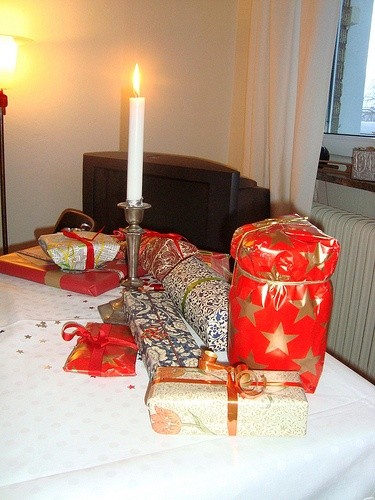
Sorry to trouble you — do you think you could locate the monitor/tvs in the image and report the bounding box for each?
[83,151,271,273]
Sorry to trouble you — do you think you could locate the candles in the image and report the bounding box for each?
[125,64,146,202]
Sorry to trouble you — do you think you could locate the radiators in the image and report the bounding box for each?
[309,202,375,384]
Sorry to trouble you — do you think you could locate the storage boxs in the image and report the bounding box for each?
[116,224,199,279]
[158,254,231,353]
[36,228,122,273]
[146,367,310,437]
[124,280,208,371]
[0,240,128,297]
[227,214,340,394]
